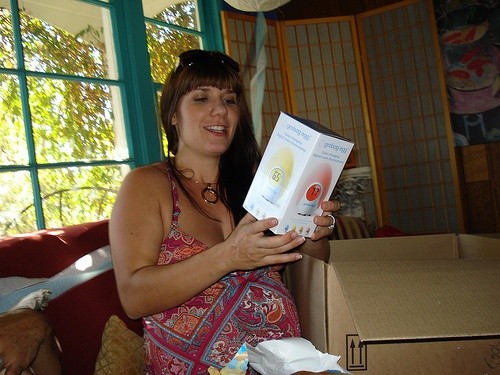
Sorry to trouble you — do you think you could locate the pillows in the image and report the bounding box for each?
[94,315,146,375]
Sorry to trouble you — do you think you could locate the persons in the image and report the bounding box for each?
[107,49,341,375]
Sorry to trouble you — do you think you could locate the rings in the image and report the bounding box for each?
[327,214,335,230]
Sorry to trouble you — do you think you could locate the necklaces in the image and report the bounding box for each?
[188,177,221,204]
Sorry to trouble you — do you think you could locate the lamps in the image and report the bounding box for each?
[224,0,289,13]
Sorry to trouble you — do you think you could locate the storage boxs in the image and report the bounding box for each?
[289,233,499,375]
[242,112,357,238]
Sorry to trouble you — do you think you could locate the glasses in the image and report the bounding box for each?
[174,49,241,81]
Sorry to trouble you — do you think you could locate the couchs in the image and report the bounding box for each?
[0,219,120,375]
